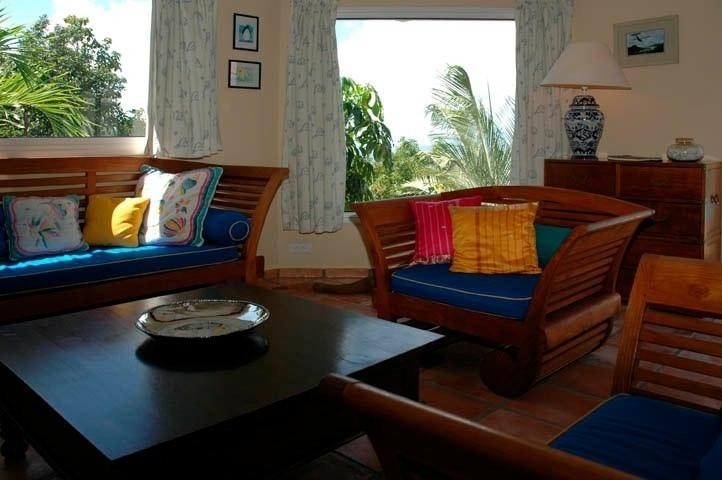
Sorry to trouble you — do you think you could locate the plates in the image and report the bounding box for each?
[134,298,273,341]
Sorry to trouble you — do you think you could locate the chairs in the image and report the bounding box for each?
[352,186,656,399]
[319,253,722,480]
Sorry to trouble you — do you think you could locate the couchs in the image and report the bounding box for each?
[0,157,289,325]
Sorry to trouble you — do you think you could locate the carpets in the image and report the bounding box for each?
[0,440,380,480]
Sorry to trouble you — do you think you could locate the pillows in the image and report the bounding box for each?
[3,163,249,262]
[409,196,572,274]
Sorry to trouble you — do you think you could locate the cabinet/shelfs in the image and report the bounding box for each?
[544,159,722,317]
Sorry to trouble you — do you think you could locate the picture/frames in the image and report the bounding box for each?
[229,59,261,89]
[613,15,679,68]
[233,13,259,52]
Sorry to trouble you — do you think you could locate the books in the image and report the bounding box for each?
[608,154,663,163]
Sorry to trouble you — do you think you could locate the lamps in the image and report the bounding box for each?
[541,41,631,161]
